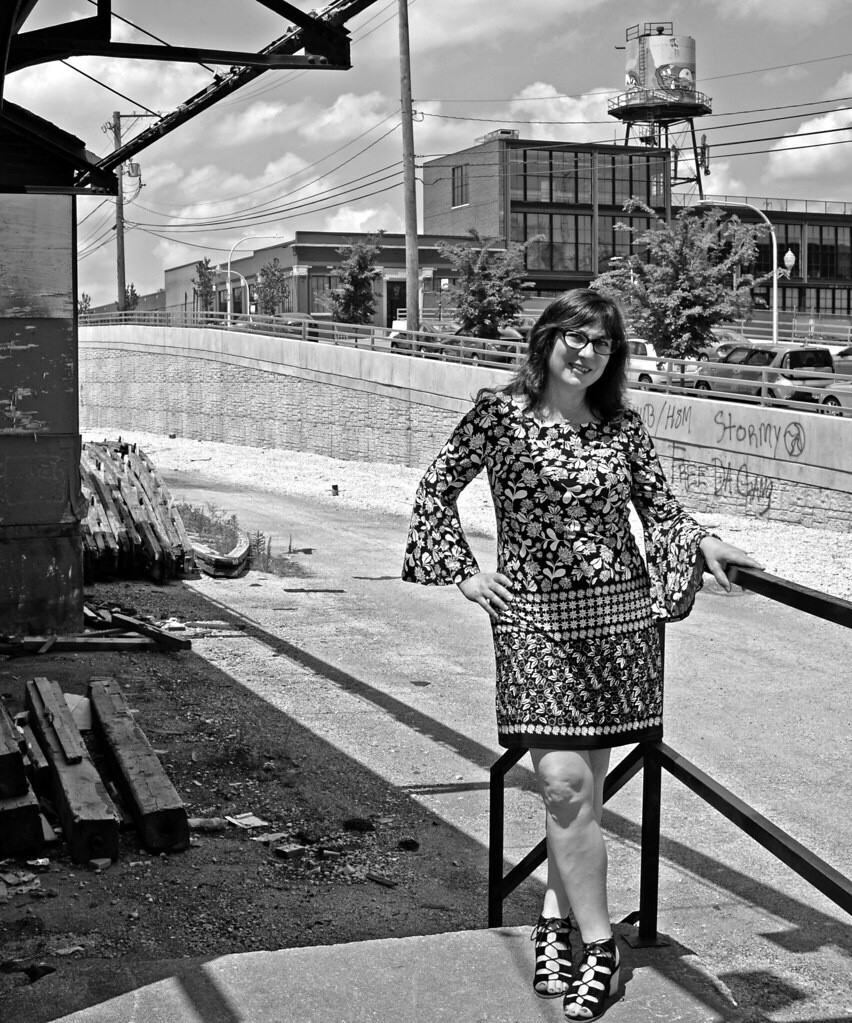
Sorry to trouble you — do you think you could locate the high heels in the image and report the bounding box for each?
[562,937,620,1022]
[532,911,572,999]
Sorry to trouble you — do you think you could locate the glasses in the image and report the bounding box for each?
[555,328,622,355]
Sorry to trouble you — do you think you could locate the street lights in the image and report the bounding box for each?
[696,198,795,344]
[228,233,285,324]
[208,263,250,327]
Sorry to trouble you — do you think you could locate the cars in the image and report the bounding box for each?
[624,328,852,417]
[232,312,321,342]
[392,318,538,371]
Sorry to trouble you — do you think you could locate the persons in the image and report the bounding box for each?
[401,288,766,1023]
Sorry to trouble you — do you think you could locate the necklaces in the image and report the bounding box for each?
[556,402,585,425]
